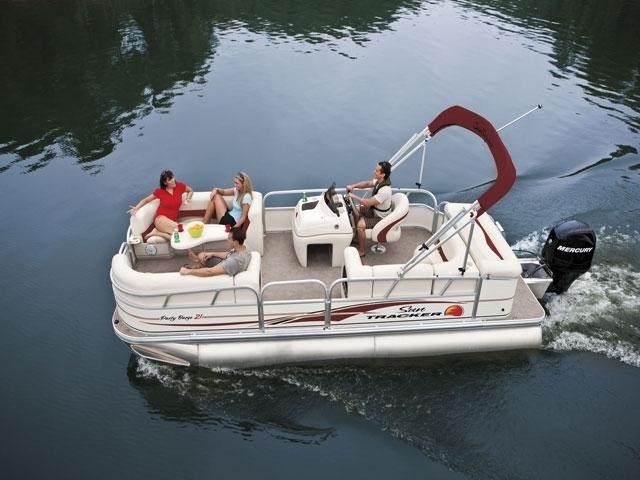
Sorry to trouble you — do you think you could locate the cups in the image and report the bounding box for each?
[178,224,183,232]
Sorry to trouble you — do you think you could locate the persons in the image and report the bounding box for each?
[203,171,252,227]
[346,161,392,257]
[126,170,200,263]
[180,227,250,277]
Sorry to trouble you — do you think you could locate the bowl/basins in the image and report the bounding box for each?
[187,221,204,238]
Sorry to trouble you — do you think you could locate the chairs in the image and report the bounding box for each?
[364,192,410,255]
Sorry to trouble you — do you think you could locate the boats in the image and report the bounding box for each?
[109,103,596,372]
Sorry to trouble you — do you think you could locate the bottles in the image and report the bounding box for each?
[173,229,180,243]
[302,192,308,202]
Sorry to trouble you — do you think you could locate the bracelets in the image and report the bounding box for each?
[186,197,190,200]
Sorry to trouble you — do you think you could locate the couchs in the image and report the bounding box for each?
[110,190,264,302]
[338,229,480,298]
